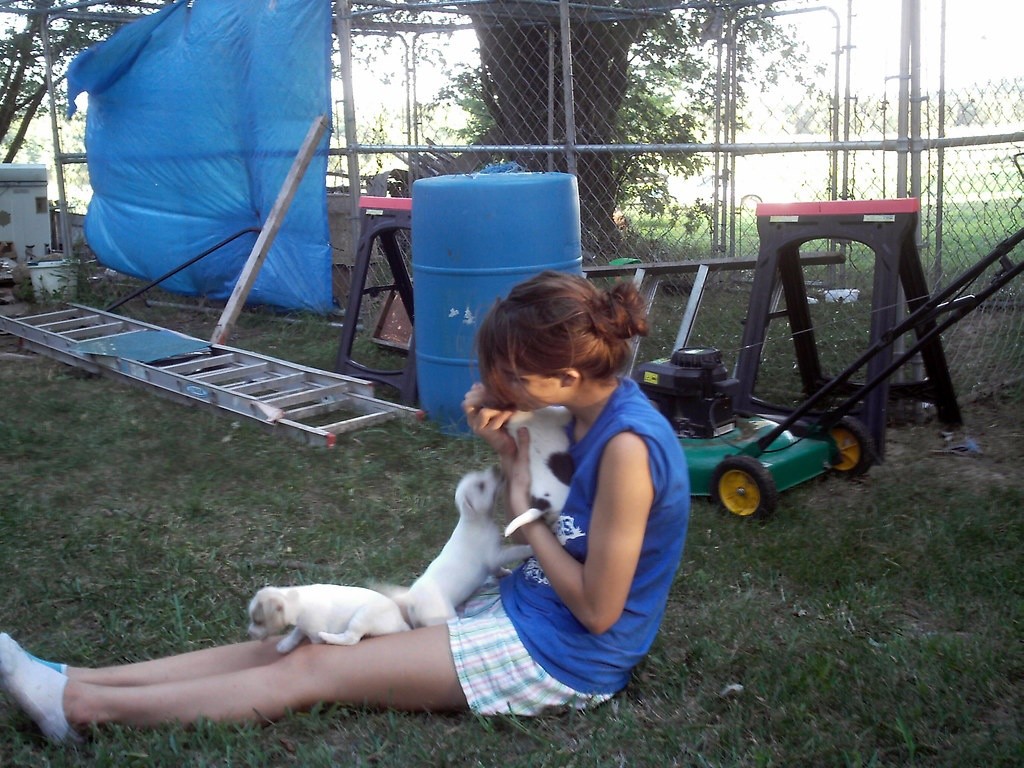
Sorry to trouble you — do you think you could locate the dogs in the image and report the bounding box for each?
[24,245,37,261]
[368,464,533,628]
[246,583,411,653]
[464,379,573,537]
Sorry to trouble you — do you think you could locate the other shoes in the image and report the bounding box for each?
[0,631,85,749]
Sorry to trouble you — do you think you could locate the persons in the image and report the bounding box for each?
[1,268,692,750]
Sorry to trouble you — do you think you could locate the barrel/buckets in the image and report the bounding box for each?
[413,172,581,436]
[28,260,77,303]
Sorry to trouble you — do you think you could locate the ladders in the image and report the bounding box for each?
[0,301,424,448]
[368,252,847,435]
